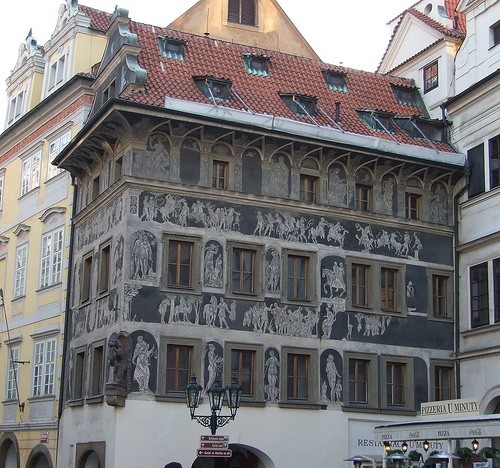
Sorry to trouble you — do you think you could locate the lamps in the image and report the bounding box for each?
[423,440,429,453]
[402,440,408,454]
[384,441,390,452]
[472,439,479,453]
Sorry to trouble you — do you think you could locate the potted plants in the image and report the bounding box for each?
[408,450,424,465]
[478,446,500,462]
[457,447,473,458]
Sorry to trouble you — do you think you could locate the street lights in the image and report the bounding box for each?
[184,370,241,468]
